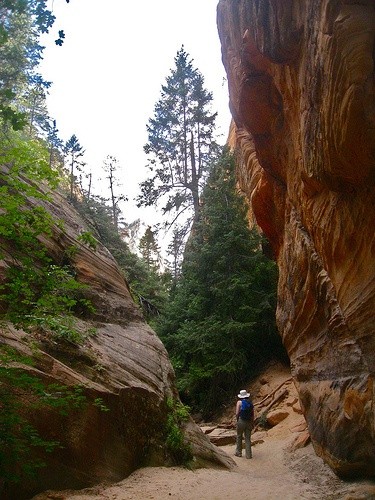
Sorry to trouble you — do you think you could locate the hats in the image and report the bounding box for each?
[237,390,250,397]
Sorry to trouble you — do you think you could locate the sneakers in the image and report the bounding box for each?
[234,452,242,457]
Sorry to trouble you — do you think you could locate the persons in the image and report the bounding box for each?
[234,390,254,459]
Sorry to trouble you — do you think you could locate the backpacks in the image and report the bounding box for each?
[240,401,254,420]
